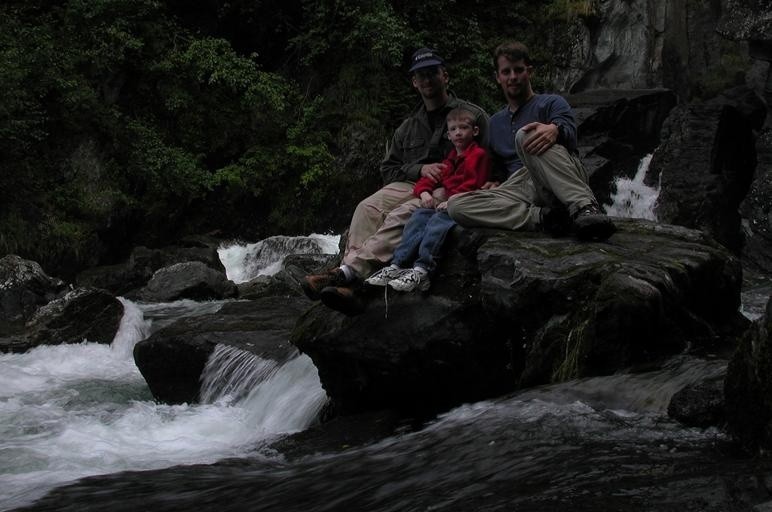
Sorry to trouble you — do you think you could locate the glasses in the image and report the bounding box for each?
[413,69,438,80]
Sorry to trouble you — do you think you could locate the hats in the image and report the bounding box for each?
[408,48,445,73]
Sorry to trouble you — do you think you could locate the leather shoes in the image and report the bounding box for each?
[300,266,360,316]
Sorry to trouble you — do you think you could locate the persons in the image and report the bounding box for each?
[362,106,492,295]
[301,46,490,318]
[446,38,618,243]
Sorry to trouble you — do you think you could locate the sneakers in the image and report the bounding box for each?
[363,266,431,292]
[575,204,611,237]
